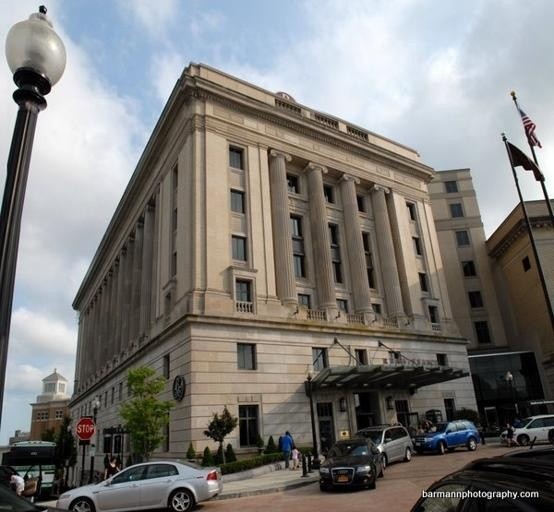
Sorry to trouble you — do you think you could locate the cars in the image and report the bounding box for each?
[319,436,384,492]
[500,414,554,447]
[0,458,224,512]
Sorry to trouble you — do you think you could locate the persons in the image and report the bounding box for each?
[281,431,292,468]
[8,470,25,497]
[505,421,521,448]
[103,456,121,480]
[292,447,301,469]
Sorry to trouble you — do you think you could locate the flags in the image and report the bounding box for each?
[505,139,545,182]
[519,105,542,148]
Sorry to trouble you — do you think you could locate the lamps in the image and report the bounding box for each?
[387,396,394,410]
[339,398,347,412]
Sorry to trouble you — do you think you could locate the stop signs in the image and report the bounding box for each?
[76,418,95,442]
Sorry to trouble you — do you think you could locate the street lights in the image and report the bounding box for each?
[0,3,68,430]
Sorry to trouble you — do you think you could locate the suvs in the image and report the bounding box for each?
[352,423,414,470]
[411,418,482,455]
[409,436,554,511]
